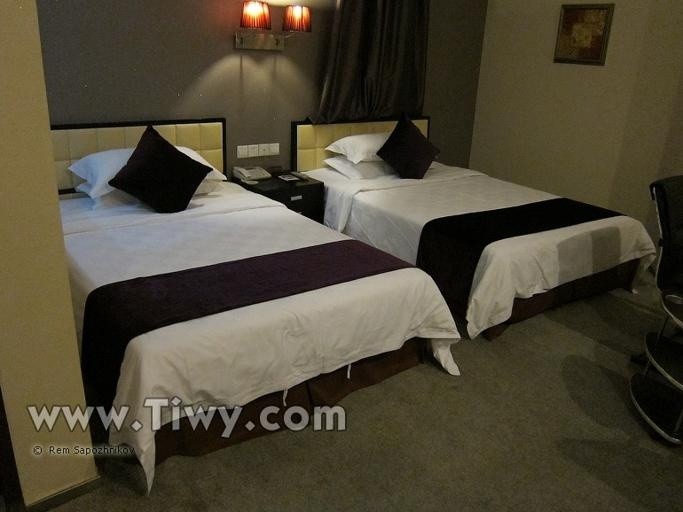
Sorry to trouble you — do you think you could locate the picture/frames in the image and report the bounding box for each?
[551,3,613,68]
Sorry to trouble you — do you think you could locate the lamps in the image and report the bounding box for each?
[239,2,313,50]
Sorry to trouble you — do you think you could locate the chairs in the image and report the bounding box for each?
[627,171,682,449]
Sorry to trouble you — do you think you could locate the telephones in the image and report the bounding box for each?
[232,166,272,185]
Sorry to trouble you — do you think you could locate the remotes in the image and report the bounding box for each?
[291,170,308,182]
